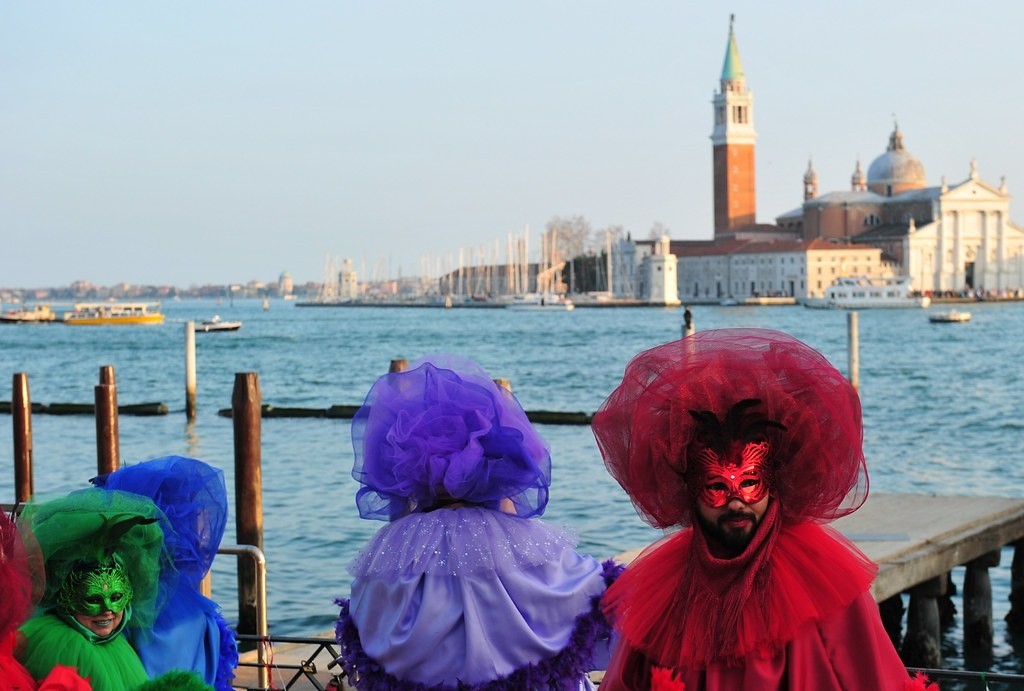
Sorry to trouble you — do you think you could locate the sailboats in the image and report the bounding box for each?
[433,230,576,313]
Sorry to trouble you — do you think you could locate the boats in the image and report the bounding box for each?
[194,320,243,332]
[61,298,166,326]
[802,272,931,311]
[928,308,973,324]
[0,301,56,323]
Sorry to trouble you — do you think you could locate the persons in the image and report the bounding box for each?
[88,456,239,691]
[584,331,940,691]
[15,487,217,691]
[333,354,627,690]
[684,305,692,329]
